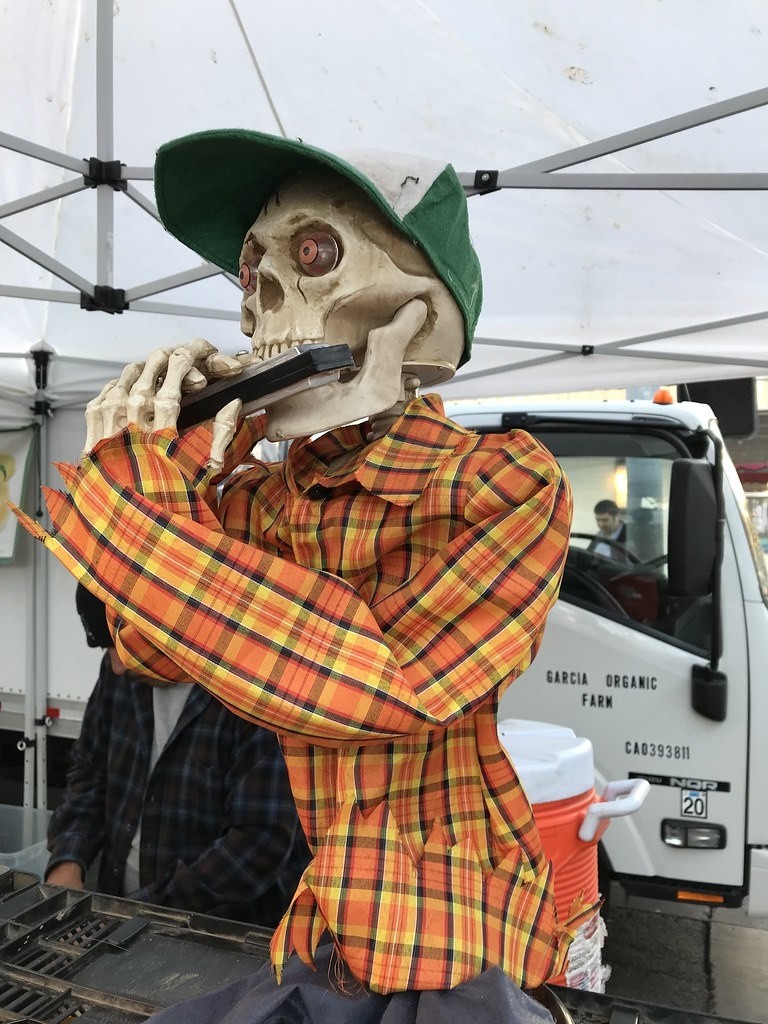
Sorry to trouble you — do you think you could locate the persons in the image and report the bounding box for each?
[43,569,313,929]
[586,500,627,562]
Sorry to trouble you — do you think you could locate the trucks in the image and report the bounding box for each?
[1,384,767,926]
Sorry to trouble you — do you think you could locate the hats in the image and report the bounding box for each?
[151,127,491,365]
